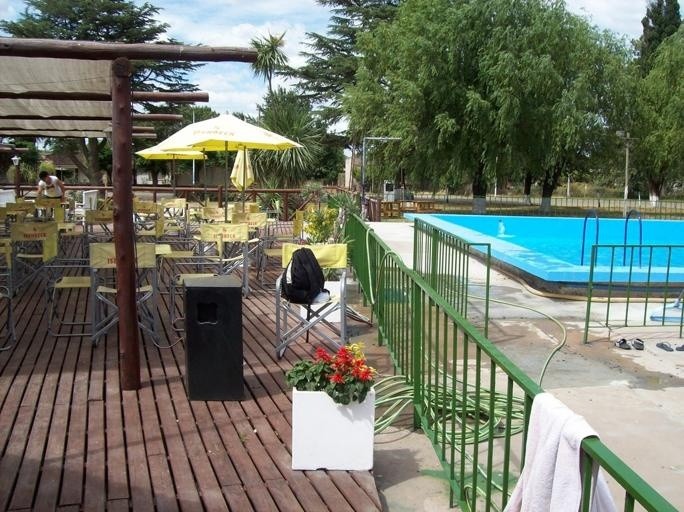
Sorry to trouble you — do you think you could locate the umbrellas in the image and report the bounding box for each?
[160,110,305,265]
[134,145,208,216]
[230,149,254,192]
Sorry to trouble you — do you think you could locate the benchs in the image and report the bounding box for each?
[380,200,442,219]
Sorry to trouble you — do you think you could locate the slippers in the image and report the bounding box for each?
[632,339,644,350]
[676,344,684,351]
[615,338,630,349]
[656,342,673,351]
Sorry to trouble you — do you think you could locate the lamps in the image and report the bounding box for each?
[11,155,22,165]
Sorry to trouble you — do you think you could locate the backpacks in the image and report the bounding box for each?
[281,248,331,343]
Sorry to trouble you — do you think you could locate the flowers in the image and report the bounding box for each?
[285,341,378,405]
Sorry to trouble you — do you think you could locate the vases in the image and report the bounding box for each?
[292,386,375,470]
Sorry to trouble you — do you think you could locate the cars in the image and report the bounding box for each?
[665,179,684,197]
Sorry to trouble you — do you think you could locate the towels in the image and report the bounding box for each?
[503,393,617,512]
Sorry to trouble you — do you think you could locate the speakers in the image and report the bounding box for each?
[181,276,243,401]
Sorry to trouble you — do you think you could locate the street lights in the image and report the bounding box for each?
[367,143,379,194]
[11,153,23,200]
[615,129,630,200]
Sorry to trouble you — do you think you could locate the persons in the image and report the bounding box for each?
[37,171,65,216]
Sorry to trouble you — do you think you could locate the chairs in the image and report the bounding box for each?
[261,203,347,360]
[2,194,274,351]
